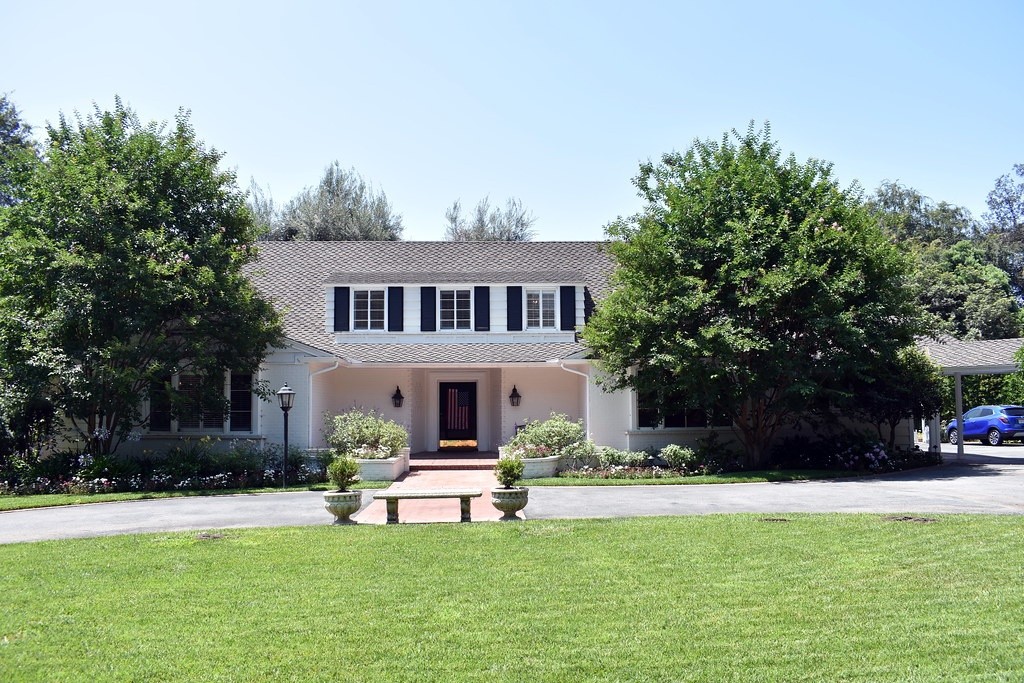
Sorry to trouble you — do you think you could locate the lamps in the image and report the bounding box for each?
[391,385,404,408]
[509,385,522,406]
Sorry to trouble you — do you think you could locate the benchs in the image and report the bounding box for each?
[372,490,482,524]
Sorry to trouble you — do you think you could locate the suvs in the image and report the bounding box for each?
[946,405,1024,445]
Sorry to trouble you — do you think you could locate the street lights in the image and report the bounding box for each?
[276,381,296,488]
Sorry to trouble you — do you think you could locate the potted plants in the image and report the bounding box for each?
[490,455,529,520]
[323,454,363,525]
